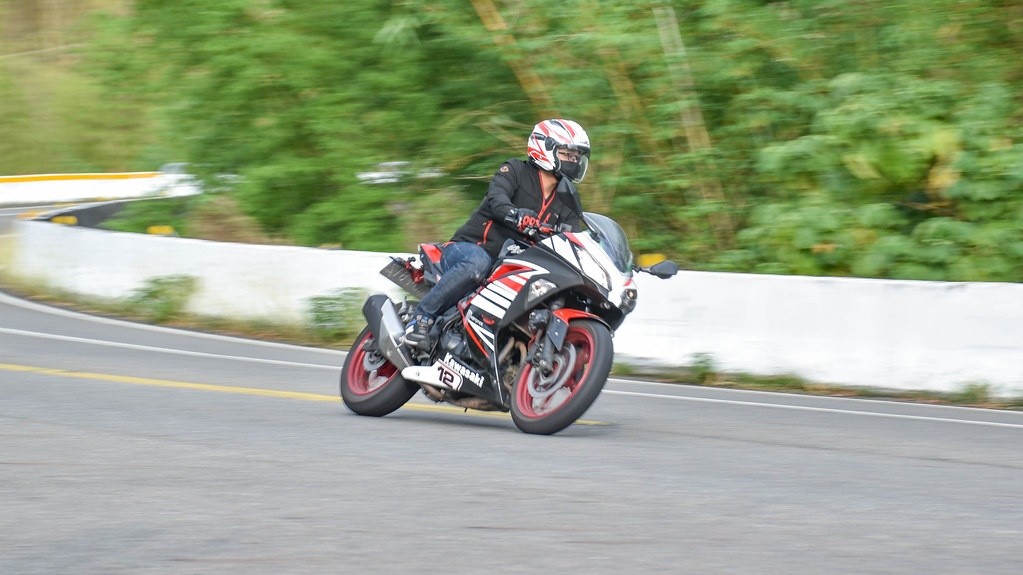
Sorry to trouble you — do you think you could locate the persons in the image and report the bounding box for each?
[403,119,591,352]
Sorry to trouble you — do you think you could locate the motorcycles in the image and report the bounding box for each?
[339,177,681,436]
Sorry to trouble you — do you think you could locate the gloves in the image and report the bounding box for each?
[504,207,541,237]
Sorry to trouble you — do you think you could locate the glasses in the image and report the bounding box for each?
[558,151,582,165]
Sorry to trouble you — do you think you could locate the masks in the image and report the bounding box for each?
[553,160,580,182]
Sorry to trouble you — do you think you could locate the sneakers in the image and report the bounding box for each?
[404,314,433,351]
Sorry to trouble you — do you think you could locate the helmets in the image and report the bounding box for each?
[528,119,590,184]
